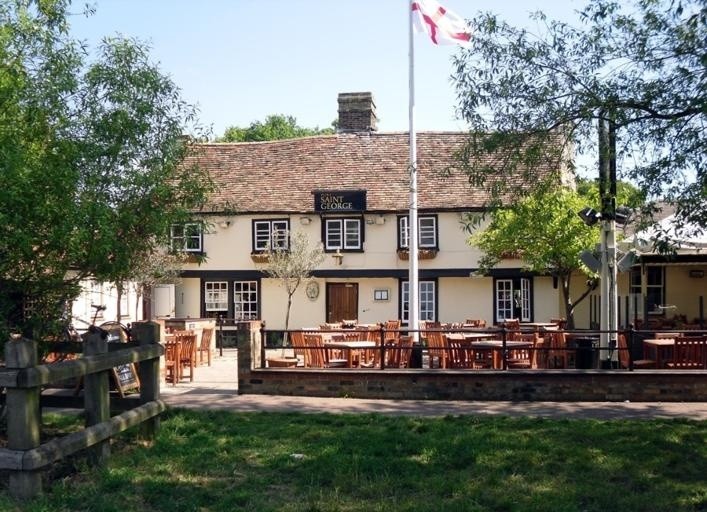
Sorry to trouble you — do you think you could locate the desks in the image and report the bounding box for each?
[578,191,636,371]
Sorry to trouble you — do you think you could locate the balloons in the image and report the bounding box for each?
[305,281,319,298]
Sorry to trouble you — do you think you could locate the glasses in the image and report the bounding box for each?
[300,217,312,225]
[218,221,229,227]
[375,216,385,225]
[332,247,343,265]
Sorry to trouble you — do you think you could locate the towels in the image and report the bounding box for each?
[406,342,423,368]
[574,337,600,369]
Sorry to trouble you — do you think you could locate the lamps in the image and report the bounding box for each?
[165,327,214,386]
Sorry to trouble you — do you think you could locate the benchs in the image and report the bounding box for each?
[412,1,479,47]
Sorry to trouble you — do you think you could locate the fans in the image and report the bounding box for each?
[99,325,140,392]
